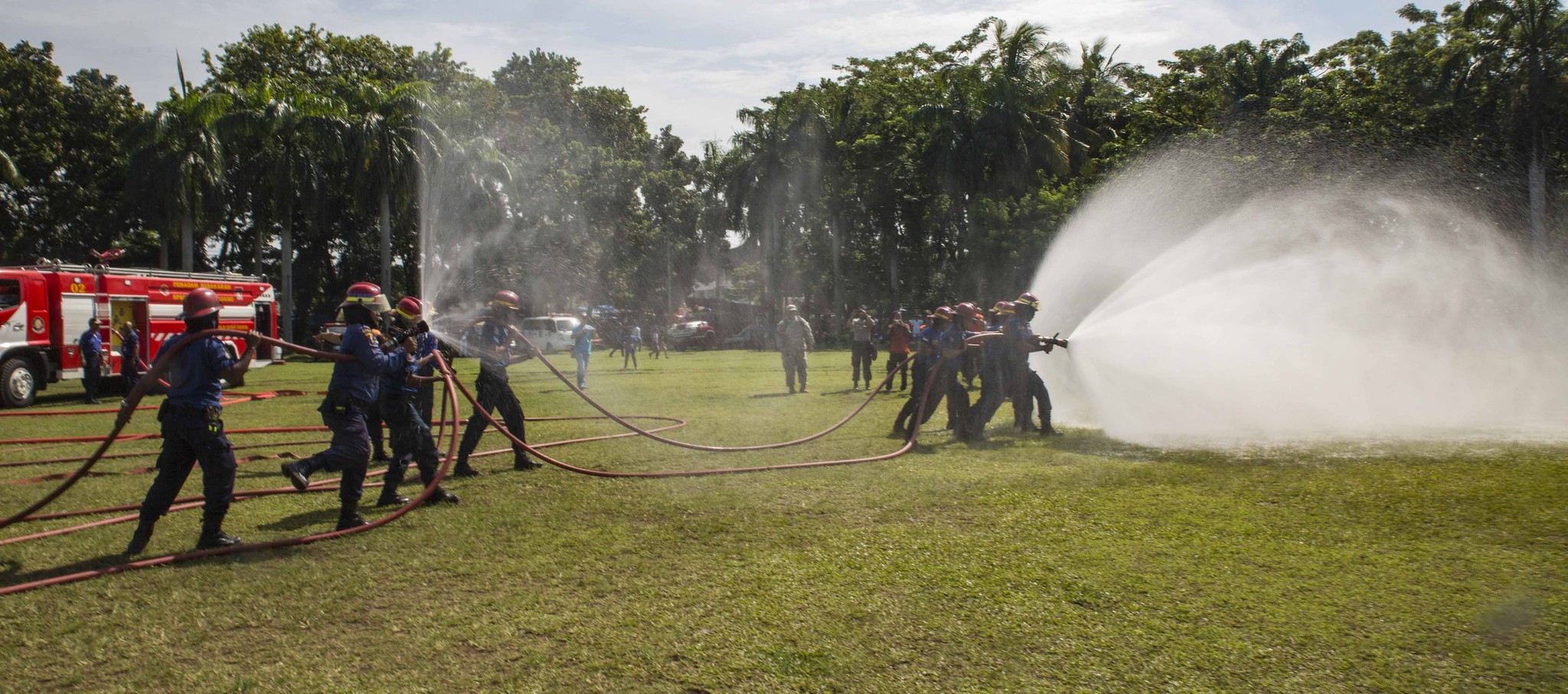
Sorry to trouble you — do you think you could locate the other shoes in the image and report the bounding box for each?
[87,399,102,404]
[853,386,858,390]
[515,454,542,470]
[376,492,409,505]
[279,460,310,490]
[865,385,871,389]
[129,532,148,553]
[373,451,392,461]
[454,463,479,476]
[789,389,796,394]
[195,529,242,548]
[336,513,367,530]
[425,490,459,504]
[888,419,1063,447]
[579,385,586,390]
[800,387,808,392]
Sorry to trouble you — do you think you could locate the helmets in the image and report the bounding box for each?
[419,299,438,315]
[388,296,423,320]
[176,288,224,320]
[488,290,520,310]
[926,292,1038,322]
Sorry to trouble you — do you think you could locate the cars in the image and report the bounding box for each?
[324,323,347,361]
[723,325,774,350]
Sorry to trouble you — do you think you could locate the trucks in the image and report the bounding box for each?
[667,306,717,351]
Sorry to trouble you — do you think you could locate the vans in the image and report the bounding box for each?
[518,312,581,353]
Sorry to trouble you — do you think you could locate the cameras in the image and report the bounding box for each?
[588,329,592,331]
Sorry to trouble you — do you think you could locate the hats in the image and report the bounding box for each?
[786,305,797,311]
[581,312,587,317]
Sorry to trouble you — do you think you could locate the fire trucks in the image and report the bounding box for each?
[0,248,290,409]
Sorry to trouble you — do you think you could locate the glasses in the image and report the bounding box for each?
[337,282,384,310]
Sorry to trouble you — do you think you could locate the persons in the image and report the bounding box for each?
[751,291,1065,441]
[74,277,668,562]
